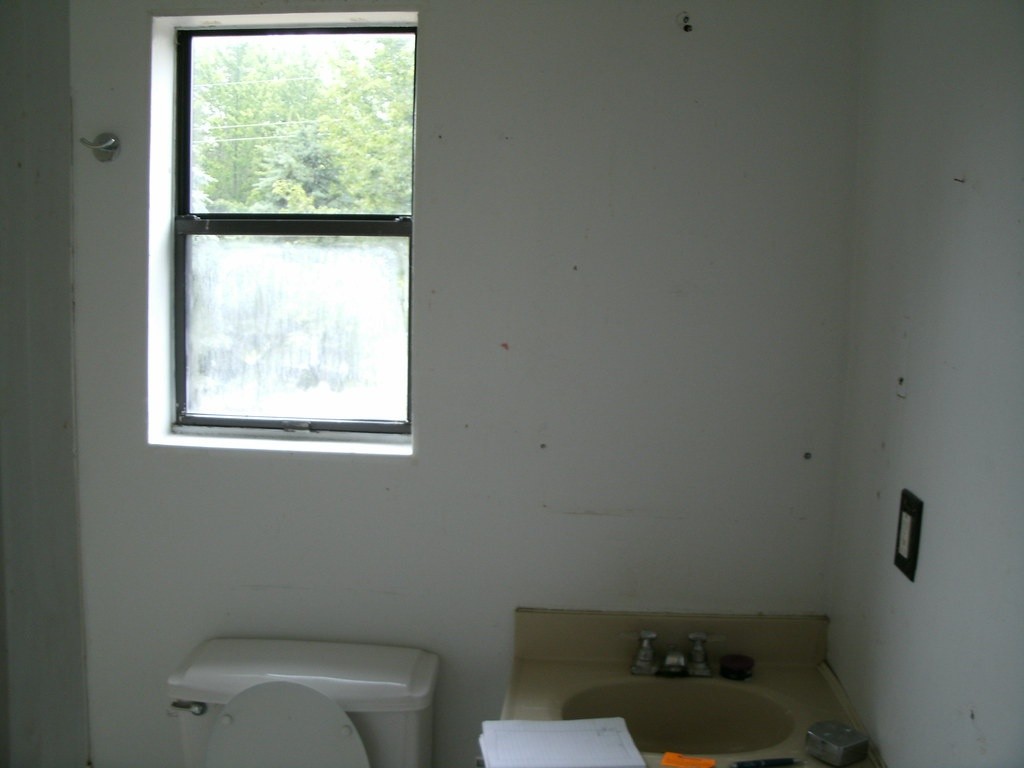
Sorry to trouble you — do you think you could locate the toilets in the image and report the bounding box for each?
[166,637,442,768]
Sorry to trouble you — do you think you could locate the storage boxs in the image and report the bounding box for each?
[805,719,871,768]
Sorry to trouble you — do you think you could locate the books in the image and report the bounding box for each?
[480,717,646,768]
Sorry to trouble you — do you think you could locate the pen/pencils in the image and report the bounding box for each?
[731,758,804,768]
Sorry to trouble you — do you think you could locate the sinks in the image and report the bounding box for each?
[557,677,795,756]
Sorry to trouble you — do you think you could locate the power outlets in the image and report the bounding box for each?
[891,485,923,584]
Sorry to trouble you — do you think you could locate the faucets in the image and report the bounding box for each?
[655,641,690,673]
